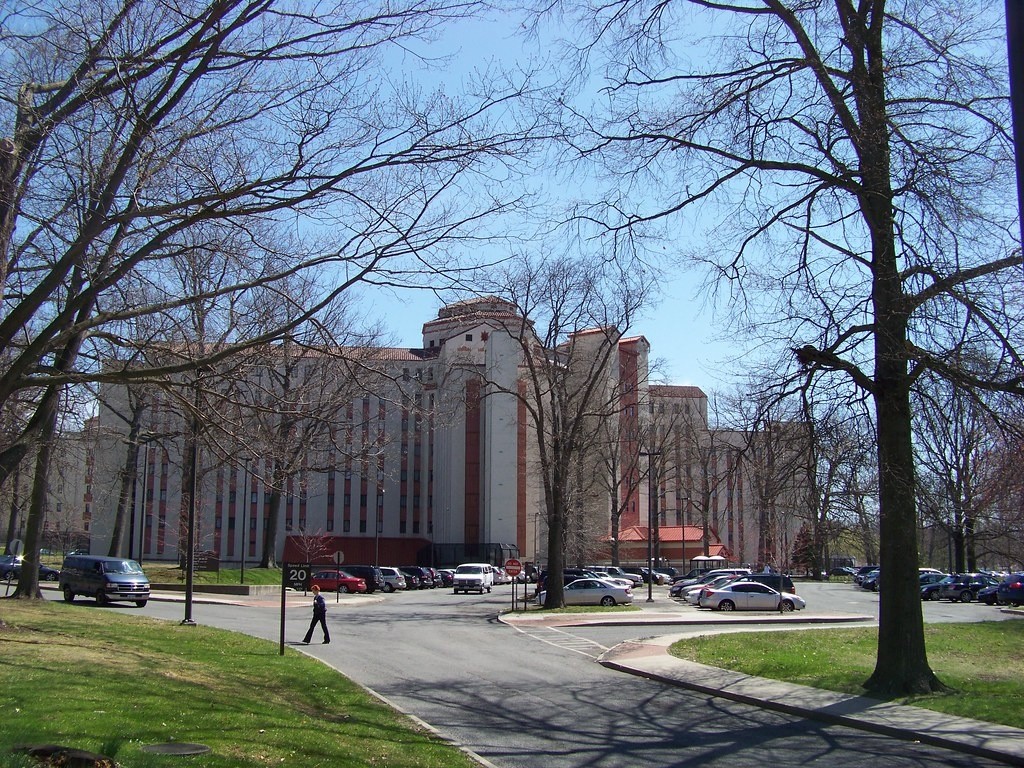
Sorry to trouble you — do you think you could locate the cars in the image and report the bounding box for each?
[39,548,53,556]
[827,566,1024,606]
[437,569,456,587]
[537,564,679,612]
[491,565,542,585]
[0,554,60,582]
[400,571,420,590]
[669,566,806,611]
[66,546,89,555]
[307,569,367,593]
[426,567,442,589]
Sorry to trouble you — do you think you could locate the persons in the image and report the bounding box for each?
[300,585,331,645]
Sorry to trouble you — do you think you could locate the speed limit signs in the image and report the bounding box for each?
[284,563,311,587]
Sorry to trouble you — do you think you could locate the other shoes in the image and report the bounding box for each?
[303,640,309,644]
[322,641,329,644]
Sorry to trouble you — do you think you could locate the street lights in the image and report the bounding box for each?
[676,498,690,576]
[639,451,662,602]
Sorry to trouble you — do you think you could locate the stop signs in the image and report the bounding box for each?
[506,560,521,575]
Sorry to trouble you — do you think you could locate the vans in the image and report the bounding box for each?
[399,566,433,589]
[339,565,385,594]
[58,554,150,608]
[379,566,406,593]
[453,563,493,595]
